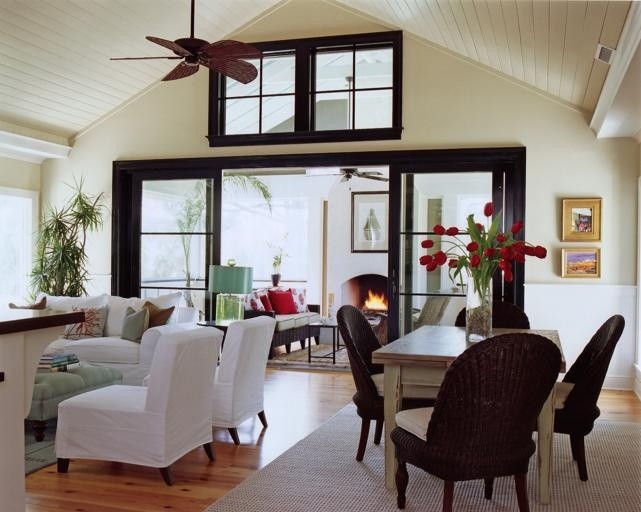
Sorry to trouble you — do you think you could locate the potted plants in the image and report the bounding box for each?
[268,247,284,287]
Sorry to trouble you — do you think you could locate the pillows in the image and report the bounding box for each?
[244,288,306,315]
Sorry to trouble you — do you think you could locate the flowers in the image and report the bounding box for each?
[417,196,548,307]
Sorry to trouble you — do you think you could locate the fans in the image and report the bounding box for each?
[305,76,390,187]
[110,0,263,84]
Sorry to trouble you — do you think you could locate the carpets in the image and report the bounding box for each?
[204,390,641,511]
[264,339,352,372]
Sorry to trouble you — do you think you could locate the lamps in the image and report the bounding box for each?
[204,260,257,325]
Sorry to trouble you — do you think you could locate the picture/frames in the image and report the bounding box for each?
[561,248,602,279]
[349,191,389,254]
[561,198,603,241]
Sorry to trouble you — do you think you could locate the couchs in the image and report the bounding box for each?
[238,287,323,362]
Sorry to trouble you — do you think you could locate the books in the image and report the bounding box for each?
[36,353,81,372]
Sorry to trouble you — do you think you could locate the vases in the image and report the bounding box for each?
[463,274,497,342]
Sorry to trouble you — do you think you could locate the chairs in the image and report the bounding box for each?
[391,335,563,511]
[454,306,531,330]
[411,293,453,330]
[524,314,627,484]
[337,303,437,462]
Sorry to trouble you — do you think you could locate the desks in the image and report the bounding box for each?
[198,319,233,340]
[368,326,570,509]
[0,304,88,510]
